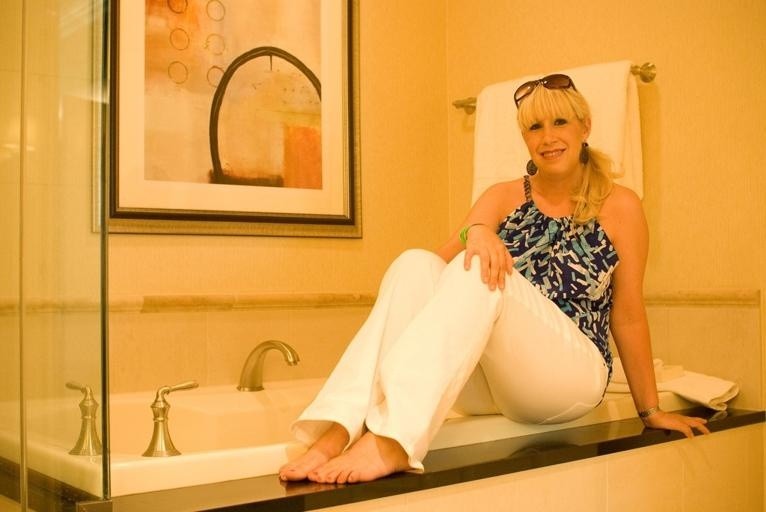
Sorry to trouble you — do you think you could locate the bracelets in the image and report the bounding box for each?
[639,404,661,418]
[459,223,485,245]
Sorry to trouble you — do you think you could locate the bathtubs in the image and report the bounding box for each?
[0,378,702,497]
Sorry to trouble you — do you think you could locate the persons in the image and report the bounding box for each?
[279,75,711,484]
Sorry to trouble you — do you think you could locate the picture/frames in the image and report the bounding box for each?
[93,0,363,237]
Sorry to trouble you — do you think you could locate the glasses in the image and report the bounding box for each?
[514,74,577,108]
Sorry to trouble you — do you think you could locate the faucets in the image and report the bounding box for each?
[236,340,300,393]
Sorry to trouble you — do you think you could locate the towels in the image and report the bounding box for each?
[473,63,645,208]
[605,356,740,412]
